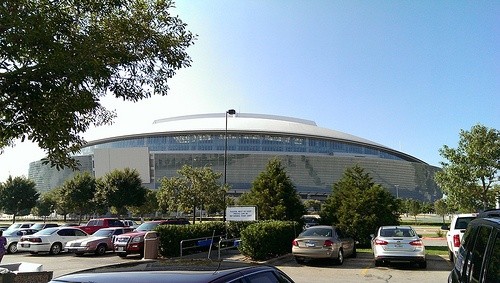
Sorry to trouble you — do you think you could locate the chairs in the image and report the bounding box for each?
[403,231,411,237]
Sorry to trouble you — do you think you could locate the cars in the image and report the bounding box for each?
[0,219,141,256]
[0,229,7,262]
[44,258,298,283]
[291,225,357,265]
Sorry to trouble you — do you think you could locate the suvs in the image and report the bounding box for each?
[111,218,191,258]
[369,224,427,269]
[447,209,500,283]
[441,213,480,263]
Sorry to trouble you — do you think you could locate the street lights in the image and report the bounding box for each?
[394,184,400,200]
[223,109,237,227]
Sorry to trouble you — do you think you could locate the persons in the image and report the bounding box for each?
[0,231,7,262]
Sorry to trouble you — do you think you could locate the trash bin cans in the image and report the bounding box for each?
[143,231,160,259]
